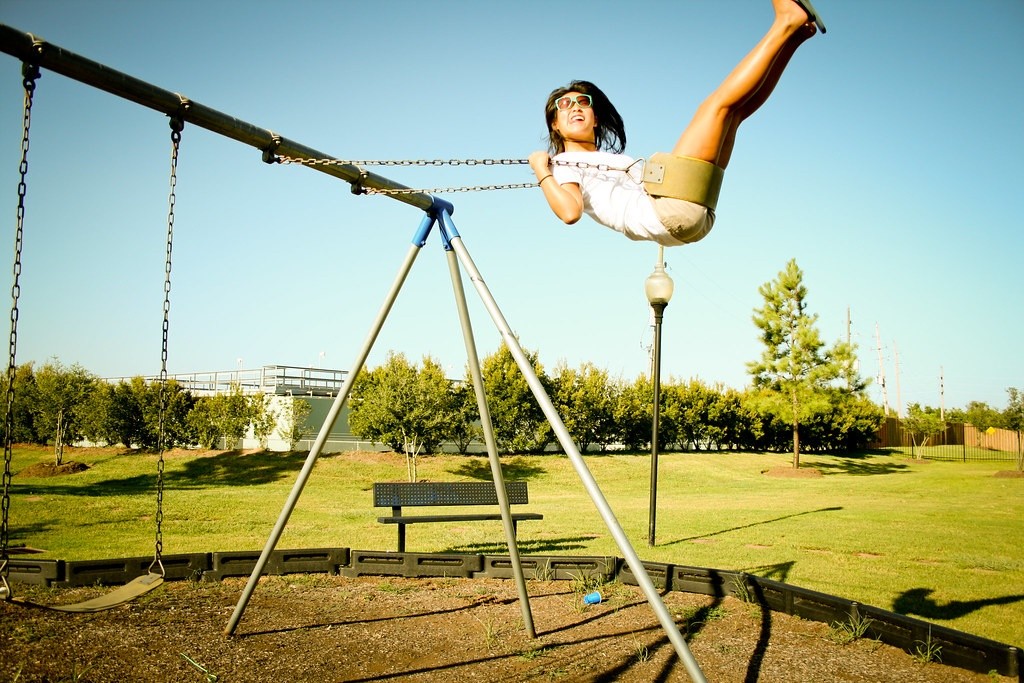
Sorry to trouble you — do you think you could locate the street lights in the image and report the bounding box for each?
[646,243,674,544]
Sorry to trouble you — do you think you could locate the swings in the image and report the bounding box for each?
[0,74,182,614]
[276,154,727,210]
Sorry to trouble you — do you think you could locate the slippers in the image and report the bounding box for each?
[814,10,826,34]
[794,0,815,22]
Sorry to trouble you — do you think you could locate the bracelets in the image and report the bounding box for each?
[539,175,552,187]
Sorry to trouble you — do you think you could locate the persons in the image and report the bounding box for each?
[528,0,825,247]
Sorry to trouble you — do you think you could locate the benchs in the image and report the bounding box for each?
[372,482,542,552]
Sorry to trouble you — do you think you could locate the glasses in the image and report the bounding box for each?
[553,94,594,111]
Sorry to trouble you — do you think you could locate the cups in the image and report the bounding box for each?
[584,592,602,604]
